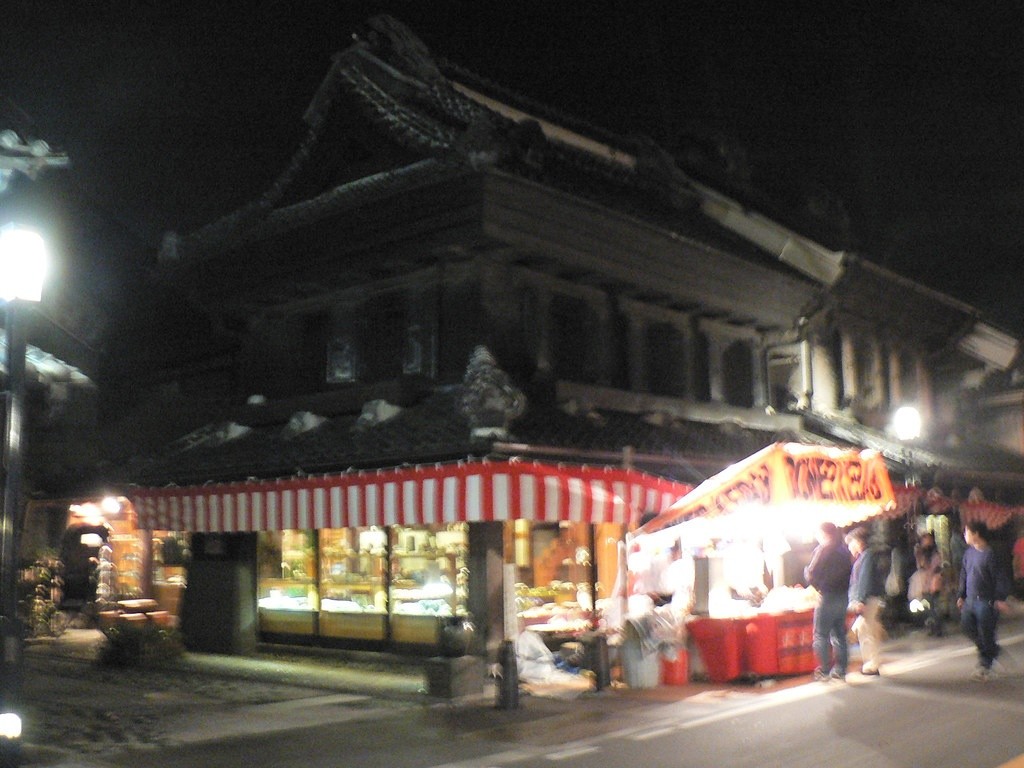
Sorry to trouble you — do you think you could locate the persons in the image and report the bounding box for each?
[1011,538,1024,581]
[844,528,885,676]
[804,522,852,683]
[914,533,943,637]
[956,521,1007,685]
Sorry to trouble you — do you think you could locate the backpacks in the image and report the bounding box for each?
[885,547,904,597]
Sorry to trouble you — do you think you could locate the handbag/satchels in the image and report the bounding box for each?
[851,612,868,636]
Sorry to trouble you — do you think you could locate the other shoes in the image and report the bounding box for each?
[828,665,846,679]
[927,623,943,638]
[860,662,880,675]
[972,665,990,683]
[813,667,833,682]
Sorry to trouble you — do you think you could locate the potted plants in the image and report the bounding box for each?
[618,620,664,689]
